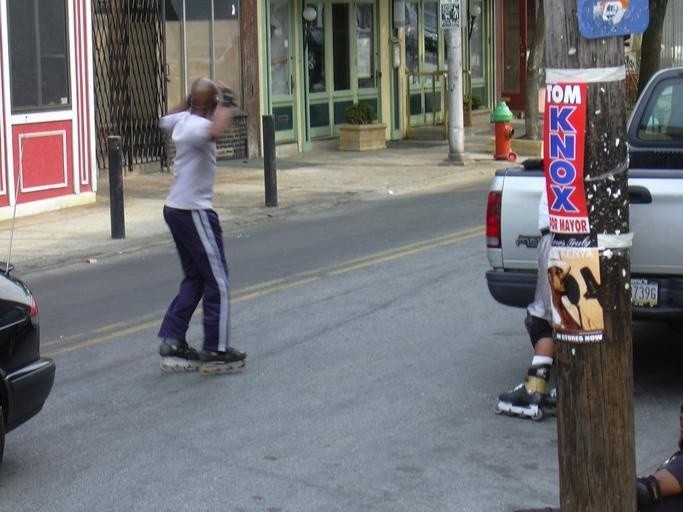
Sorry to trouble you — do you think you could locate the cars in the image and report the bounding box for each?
[405,9,437,53]
[0,260,56,465]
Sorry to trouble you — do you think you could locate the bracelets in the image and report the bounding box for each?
[221,95,233,108]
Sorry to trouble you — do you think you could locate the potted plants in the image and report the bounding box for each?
[336,102,388,152]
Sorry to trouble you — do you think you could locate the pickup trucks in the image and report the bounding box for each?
[484,65,683,322]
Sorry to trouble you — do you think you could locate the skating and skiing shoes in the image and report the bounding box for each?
[158,339,247,373]
[494,362,551,421]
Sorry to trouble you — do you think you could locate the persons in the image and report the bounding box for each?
[450,3,458,23]
[497,186,555,408]
[156,75,249,364]
[634,398,682,512]
[547,249,586,332]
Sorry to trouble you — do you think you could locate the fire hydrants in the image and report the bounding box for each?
[490,101,517,161]
